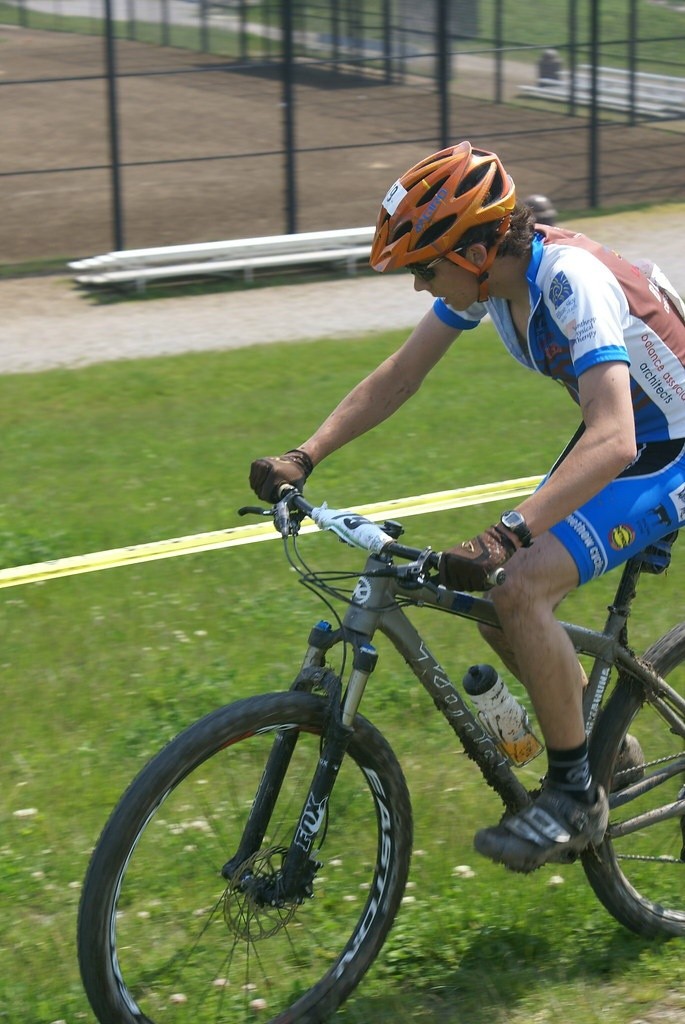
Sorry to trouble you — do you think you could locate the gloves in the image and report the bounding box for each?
[249,450,314,504]
[434,525,517,593]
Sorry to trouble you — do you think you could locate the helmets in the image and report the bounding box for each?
[368,141,516,273]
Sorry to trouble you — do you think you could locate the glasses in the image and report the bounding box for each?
[403,241,487,282]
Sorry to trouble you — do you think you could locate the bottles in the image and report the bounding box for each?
[463,664,539,763]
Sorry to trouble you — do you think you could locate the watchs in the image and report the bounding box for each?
[500,510,535,548]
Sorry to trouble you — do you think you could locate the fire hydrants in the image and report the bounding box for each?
[538,50,563,89]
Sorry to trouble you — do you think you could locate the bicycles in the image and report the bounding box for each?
[73,467,684,1024]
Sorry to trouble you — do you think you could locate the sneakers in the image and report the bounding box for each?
[474,784,610,872]
[609,732,646,792]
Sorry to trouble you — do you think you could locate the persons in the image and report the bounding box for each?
[538,48,561,87]
[249,139,685,874]
